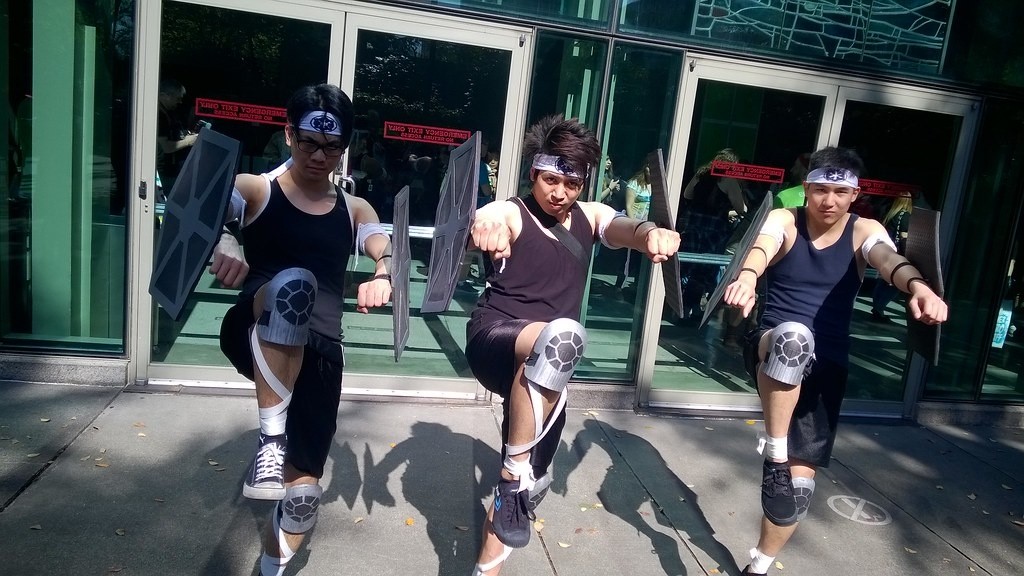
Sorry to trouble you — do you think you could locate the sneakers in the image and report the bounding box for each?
[760,457,798,528]
[742,565,768,576]
[492,477,537,548]
[242,436,289,500]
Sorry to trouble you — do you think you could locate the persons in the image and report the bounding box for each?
[209,83,393,576]
[723,147,947,576]
[155,79,914,348]
[466,113,681,576]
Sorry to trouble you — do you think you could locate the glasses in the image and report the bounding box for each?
[289,126,346,158]
